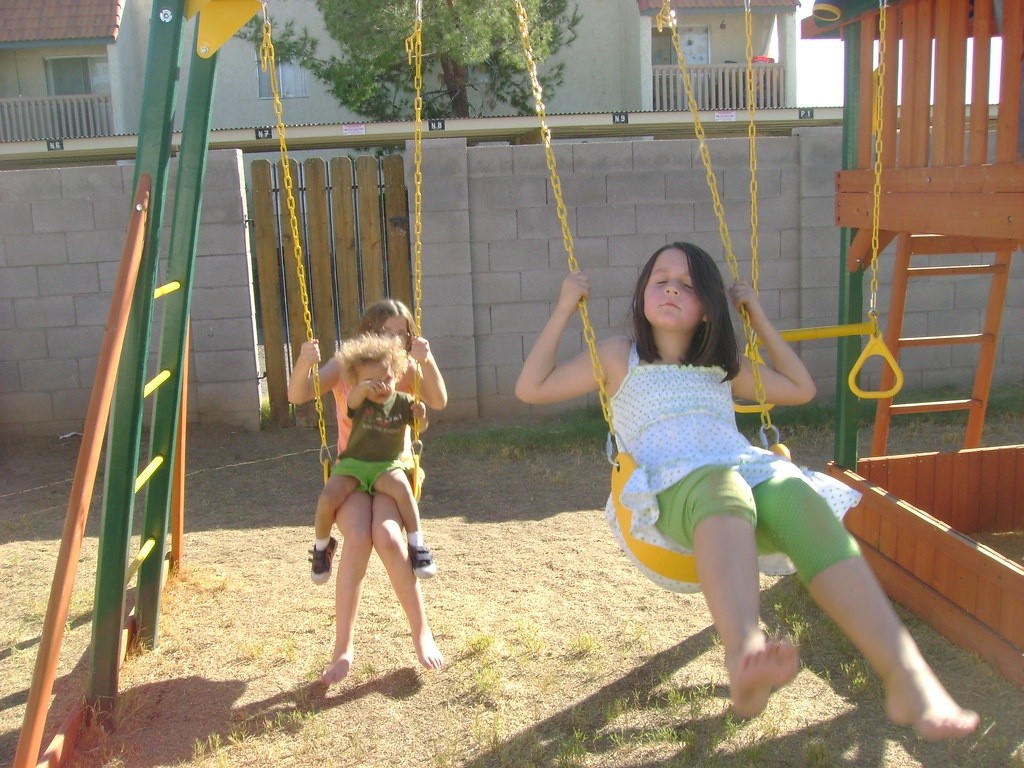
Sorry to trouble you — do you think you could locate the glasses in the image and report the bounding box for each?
[374,326,410,340]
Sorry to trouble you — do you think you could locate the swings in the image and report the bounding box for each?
[261,18,424,536]
[747,0,890,343]
[515,0,791,585]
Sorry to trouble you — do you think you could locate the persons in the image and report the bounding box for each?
[308,329,437,586]
[514,242,980,740]
[287,299,447,681]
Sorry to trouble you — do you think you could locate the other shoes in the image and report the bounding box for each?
[307,536,338,583]
[408,543,436,579]
